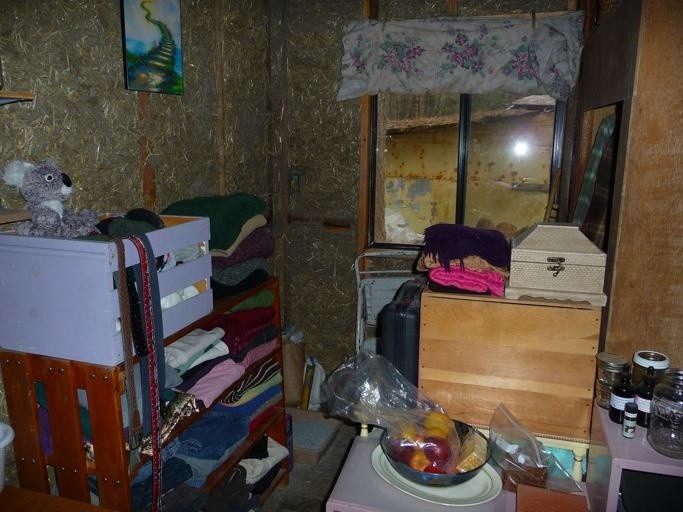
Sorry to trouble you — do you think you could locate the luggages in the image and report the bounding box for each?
[375,276,427,410]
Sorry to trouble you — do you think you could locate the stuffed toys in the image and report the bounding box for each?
[2,160,101,239]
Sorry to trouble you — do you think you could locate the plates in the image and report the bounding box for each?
[370,443,504,506]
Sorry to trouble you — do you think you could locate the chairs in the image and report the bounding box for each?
[348,244,423,367]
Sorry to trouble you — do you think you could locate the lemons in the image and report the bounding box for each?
[424,410,450,439]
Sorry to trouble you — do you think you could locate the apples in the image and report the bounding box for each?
[409,450,430,472]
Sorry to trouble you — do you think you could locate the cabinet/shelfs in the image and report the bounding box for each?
[0,269,297,512]
[319,399,681,512]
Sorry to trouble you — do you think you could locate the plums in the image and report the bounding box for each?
[390,439,414,461]
[424,437,451,462]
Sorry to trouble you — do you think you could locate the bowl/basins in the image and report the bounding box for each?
[379,418,490,485]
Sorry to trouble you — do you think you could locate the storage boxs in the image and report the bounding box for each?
[0,211,215,375]
[500,220,610,297]
[414,279,605,449]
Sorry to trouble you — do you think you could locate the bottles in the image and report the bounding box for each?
[635,366,656,429]
[594,353,630,407]
[621,402,639,438]
[646,369,682,460]
[631,350,671,389]
[609,364,636,424]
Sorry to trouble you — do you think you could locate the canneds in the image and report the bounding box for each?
[596,350,670,410]
